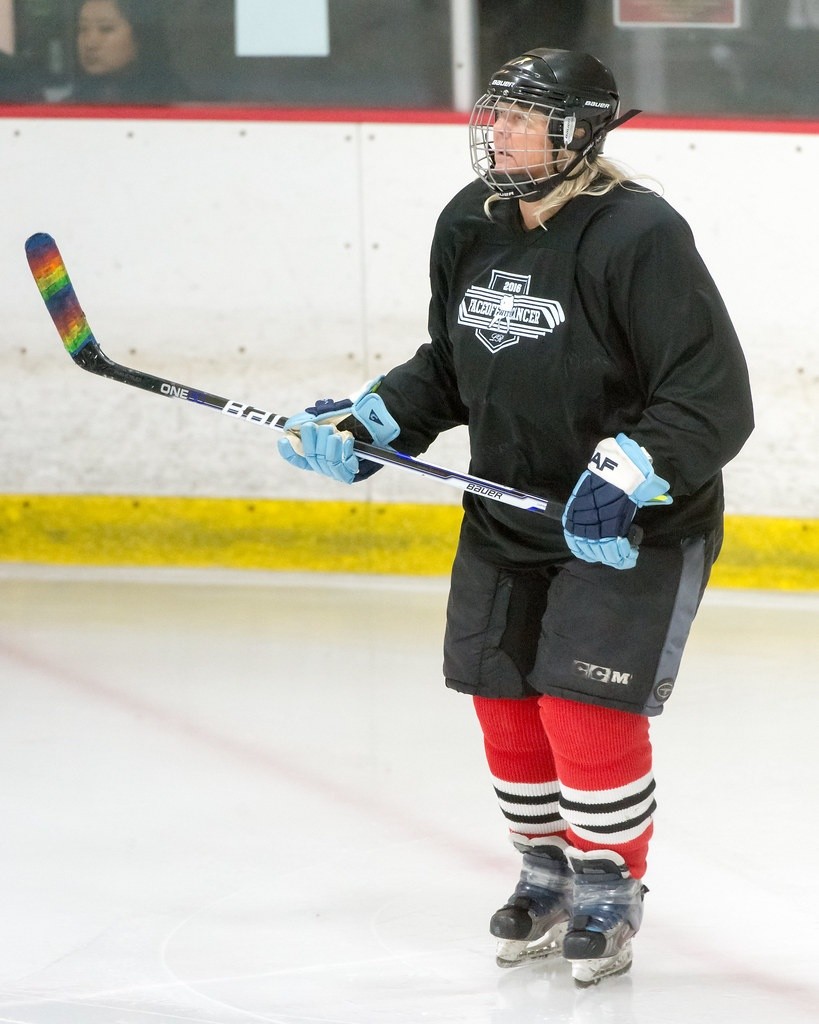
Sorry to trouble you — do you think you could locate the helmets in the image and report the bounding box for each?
[469,47,642,203]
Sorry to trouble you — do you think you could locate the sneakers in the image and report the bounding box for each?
[562,846,650,988]
[490,834,573,969]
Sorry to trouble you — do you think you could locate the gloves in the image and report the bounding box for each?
[562,433,674,570]
[277,375,401,485]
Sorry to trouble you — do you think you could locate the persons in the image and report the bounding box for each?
[52,0,197,106]
[275,46,754,985]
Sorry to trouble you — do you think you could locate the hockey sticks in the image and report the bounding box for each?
[20,226,571,525]
